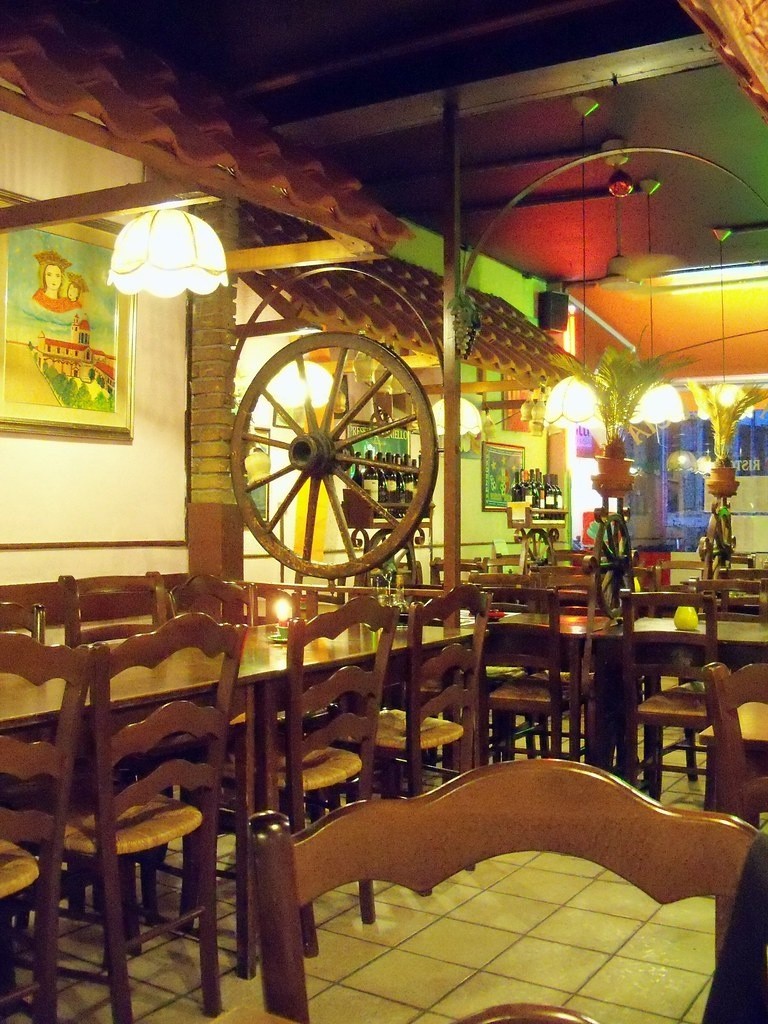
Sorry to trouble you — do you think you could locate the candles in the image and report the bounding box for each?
[277,598,287,627]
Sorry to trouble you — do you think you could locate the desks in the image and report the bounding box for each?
[589,616,768,792]
[428,610,609,761]
[244,599,346,626]
[698,700,768,831]
[0,623,490,792]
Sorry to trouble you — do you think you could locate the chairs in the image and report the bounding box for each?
[0,550,768,1024]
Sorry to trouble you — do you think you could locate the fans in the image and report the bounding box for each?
[594,140,687,302]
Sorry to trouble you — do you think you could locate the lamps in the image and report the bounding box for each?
[696,228,755,420]
[106,205,230,299]
[628,179,690,425]
[543,96,606,430]
[264,360,334,409]
[693,454,710,475]
[666,449,698,475]
[432,397,482,435]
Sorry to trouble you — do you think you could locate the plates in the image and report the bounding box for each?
[267,635,287,643]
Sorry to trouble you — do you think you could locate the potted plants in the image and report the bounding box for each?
[546,324,703,499]
[681,377,768,493]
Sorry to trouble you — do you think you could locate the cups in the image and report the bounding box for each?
[633,577,640,592]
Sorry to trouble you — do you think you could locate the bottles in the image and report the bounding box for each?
[513,468,563,519]
[353,451,419,518]
[674,606,698,630]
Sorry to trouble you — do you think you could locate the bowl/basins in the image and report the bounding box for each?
[277,627,288,638]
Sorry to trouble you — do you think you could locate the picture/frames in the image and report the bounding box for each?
[272,409,305,431]
[344,420,412,489]
[480,440,525,513]
[0,188,139,445]
[501,374,532,431]
[242,426,272,527]
[575,426,604,459]
